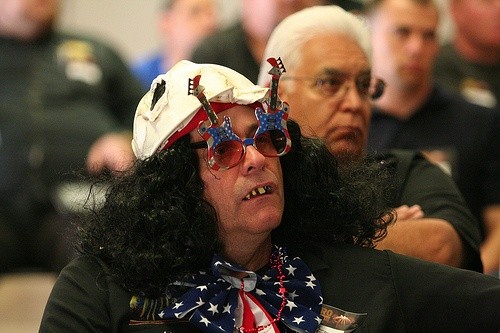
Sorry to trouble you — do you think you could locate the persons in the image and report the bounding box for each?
[0,0,146,275]
[35,57,499,333]
[367,1,499,276]
[192,1,300,84]
[443,0,497,111]
[252,6,486,276]
[127,0,222,89]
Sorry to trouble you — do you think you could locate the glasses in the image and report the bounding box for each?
[286,72,386,101]
[187,57,292,171]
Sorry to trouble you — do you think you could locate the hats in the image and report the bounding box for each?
[131,59,271,162]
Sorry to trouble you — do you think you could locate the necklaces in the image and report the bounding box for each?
[203,252,287,332]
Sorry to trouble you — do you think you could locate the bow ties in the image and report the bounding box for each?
[157,244,323,333]
